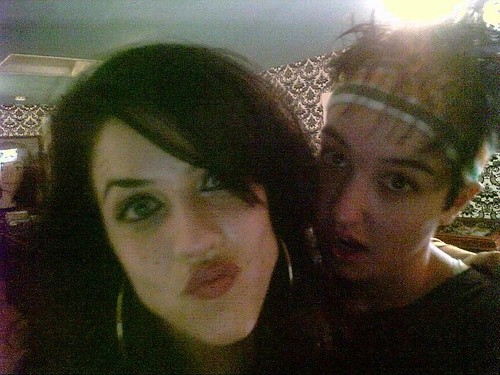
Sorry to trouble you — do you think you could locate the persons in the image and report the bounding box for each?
[0,152,49,210]
[12,42,500,375]
[292,22,500,375]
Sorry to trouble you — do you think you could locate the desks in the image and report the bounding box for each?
[434,218,500,253]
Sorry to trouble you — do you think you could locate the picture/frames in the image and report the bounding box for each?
[0,136,47,212]
